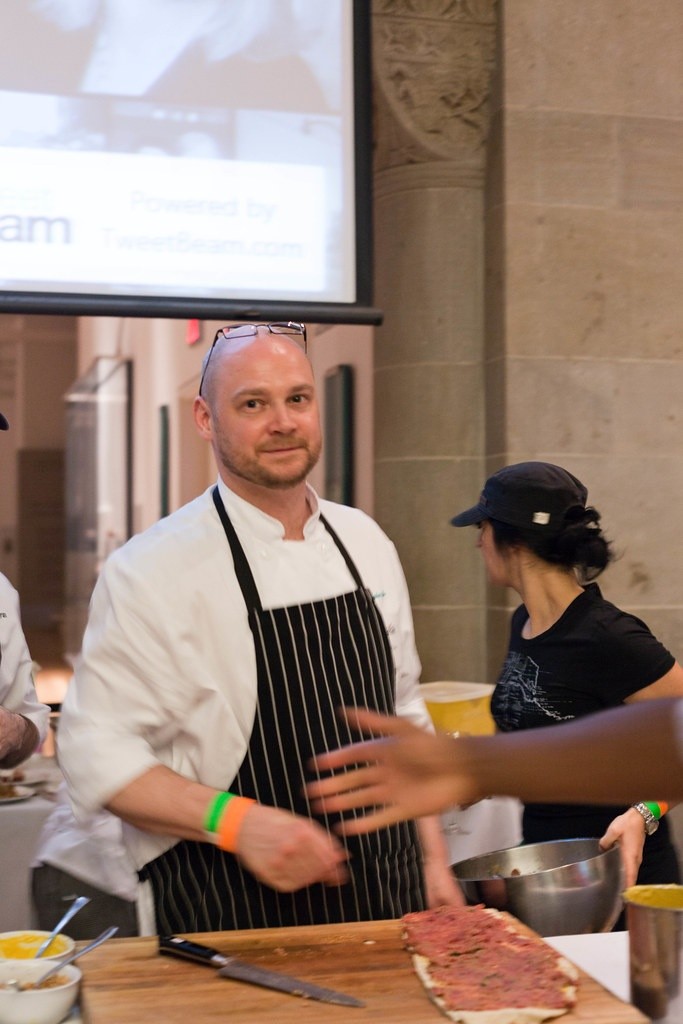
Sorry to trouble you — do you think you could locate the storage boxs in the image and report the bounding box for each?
[415,680,496,739]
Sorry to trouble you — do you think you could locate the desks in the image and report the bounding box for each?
[0,766,527,935]
[61,931,630,1024]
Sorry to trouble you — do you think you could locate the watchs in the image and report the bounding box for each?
[633,801,659,835]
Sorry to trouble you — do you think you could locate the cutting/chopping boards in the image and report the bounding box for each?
[70,910,653,1024]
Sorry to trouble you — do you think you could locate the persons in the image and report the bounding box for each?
[448,461,683,933]
[54,323,465,943]
[0,406,151,951]
[303,692,683,1024]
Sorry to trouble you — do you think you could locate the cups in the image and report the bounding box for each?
[619,883,683,1024]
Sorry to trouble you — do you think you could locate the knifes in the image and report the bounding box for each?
[156,932,368,1008]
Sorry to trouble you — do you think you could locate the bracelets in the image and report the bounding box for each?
[644,799,669,820]
[201,792,256,852]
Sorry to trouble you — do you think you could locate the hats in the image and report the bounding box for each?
[451,461,594,528]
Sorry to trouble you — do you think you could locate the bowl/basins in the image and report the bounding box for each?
[0,930,76,963]
[449,837,624,937]
[0,959,83,1024]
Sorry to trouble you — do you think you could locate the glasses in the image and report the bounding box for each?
[200,321,308,398]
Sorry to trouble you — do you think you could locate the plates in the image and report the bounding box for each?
[0,786,37,802]
[0,768,50,785]
